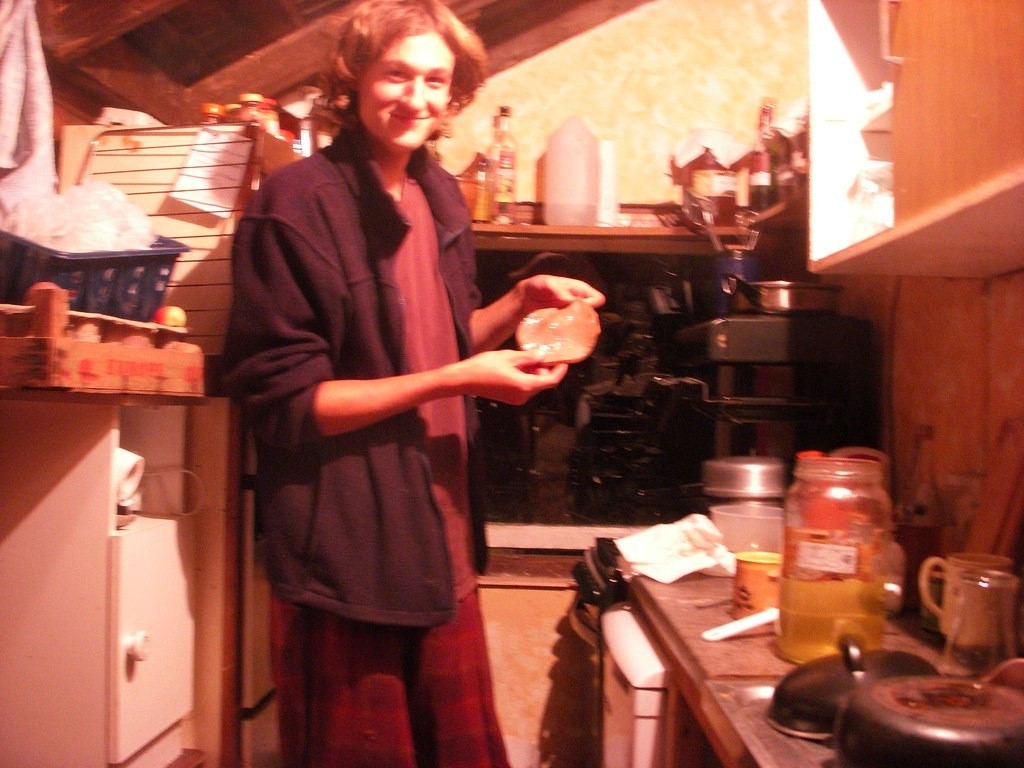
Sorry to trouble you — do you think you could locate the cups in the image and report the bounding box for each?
[919,551,1014,634]
[728,552,782,624]
[708,506,785,560]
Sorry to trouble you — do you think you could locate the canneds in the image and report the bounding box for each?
[688,129,736,226]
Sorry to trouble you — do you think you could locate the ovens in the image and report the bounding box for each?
[565,550,614,768]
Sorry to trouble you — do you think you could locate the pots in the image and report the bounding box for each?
[837,636,1024,768]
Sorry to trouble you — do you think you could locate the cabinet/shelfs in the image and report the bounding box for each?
[0,390,200,768]
[808,0,1024,277]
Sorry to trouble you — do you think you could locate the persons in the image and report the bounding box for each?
[217,0,606,768]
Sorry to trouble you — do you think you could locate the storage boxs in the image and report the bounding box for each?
[0,229,192,320]
[0,337,207,398]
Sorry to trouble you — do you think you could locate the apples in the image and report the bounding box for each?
[157,305,186,327]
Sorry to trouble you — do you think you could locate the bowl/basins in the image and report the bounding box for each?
[722,271,845,313]
[762,647,943,741]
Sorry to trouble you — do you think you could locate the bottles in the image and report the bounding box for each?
[919,570,1019,679]
[488,106,518,226]
[543,119,597,227]
